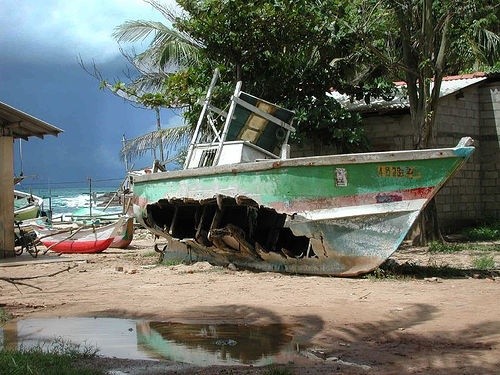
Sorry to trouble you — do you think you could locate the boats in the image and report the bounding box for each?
[130,66,478,277]
[35,224,116,256]
[14,191,43,221]
[75,215,134,251]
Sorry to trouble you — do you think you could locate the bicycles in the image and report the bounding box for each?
[11,221,38,259]
